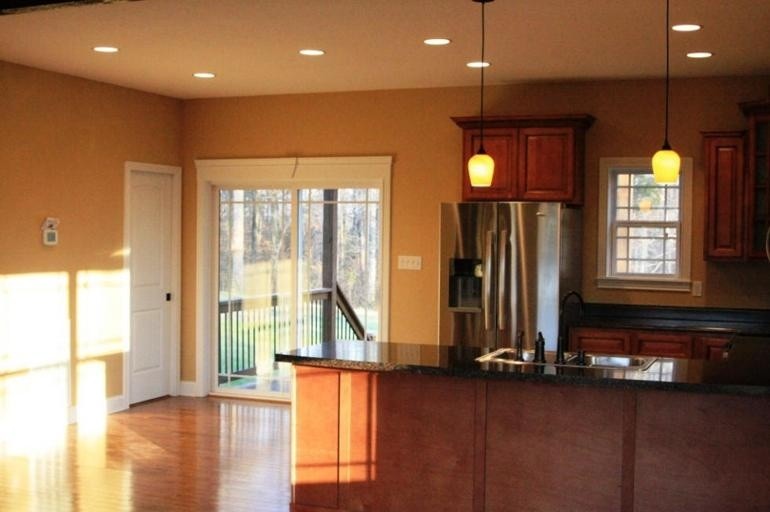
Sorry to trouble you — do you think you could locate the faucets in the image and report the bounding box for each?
[555,289,586,364]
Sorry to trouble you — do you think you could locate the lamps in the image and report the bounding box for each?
[650,1,681,186]
[467,0,495,190]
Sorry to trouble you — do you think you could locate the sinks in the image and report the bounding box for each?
[565,352,659,372]
[474,347,575,365]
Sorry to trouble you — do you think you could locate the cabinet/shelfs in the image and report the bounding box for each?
[705,137,748,257]
[463,126,586,204]
[748,115,770,259]
[572,329,734,360]
